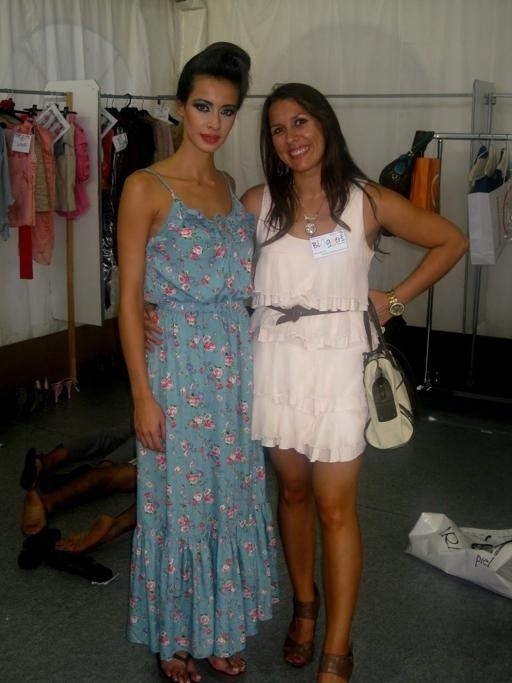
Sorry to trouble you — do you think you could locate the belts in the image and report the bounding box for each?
[258,305,350,325]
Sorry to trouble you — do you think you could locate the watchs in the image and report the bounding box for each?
[385,290,407,318]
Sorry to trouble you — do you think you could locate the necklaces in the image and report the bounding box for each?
[290,184,328,235]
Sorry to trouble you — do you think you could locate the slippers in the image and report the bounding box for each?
[156,651,200,683]
[205,654,248,677]
[17,526,114,582]
[20,447,92,494]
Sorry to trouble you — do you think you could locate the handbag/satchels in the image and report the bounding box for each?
[379,130,440,237]
[467,177,511,265]
[362,297,414,451]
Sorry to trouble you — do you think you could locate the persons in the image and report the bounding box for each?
[116,42,278,683]
[24,417,137,489]
[144,84,469,683]
[21,457,140,553]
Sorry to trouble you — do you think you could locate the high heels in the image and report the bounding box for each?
[282,582,321,668]
[22,378,74,417]
[318,641,354,683]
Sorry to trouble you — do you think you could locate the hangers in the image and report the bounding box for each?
[0,85,171,133]
[469,131,510,185]
